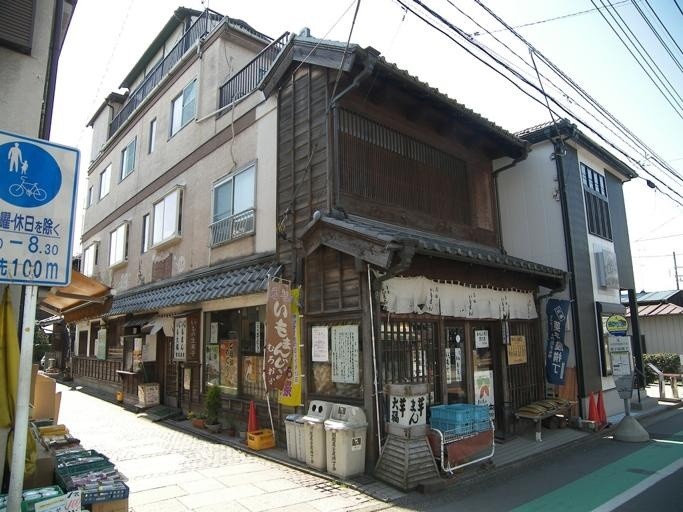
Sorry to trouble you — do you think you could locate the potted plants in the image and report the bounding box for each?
[191,382,246,437]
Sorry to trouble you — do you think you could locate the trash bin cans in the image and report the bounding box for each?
[41,357,56,370]
[285,400,368,479]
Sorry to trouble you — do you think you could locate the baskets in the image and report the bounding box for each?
[50,452,131,505]
[429,402,491,436]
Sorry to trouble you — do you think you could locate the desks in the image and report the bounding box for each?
[514,403,576,442]
